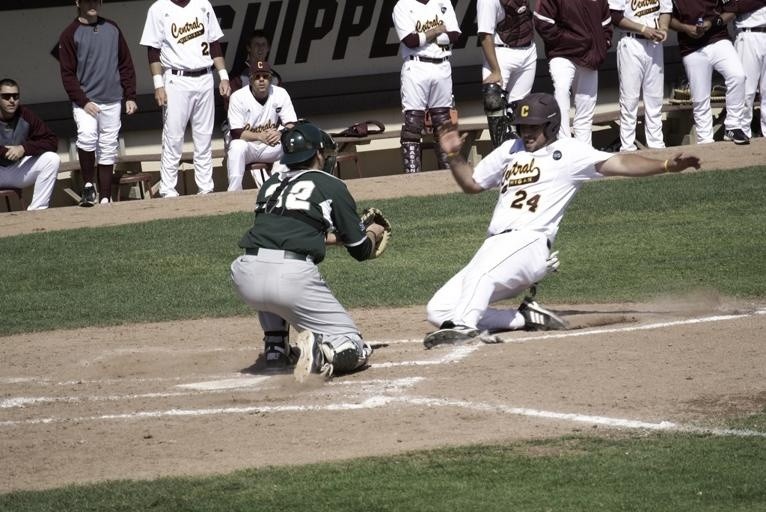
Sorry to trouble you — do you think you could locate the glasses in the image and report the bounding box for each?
[1,93,19,101]
[254,75,268,80]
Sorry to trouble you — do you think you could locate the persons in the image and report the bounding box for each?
[227,122,393,381]
[1,78,62,212]
[604,1,674,153]
[57,0,140,208]
[231,28,282,91]
[726,0,766,140]
[421,90,705,347]
[390,0,463,175]
[138,0,232,198]
[664,1,752,146]
[474,0,539,151]
[225,59,300,195]
[531,0,615,150]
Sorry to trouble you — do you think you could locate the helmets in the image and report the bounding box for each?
[511,93,561,138]
[280,123,339,174]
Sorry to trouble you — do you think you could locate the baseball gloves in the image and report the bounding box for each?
[357,208,395,258]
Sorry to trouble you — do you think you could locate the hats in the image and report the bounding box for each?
[249,62,270,72]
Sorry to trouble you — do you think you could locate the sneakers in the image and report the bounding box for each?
[518,296,566,330]
[723,130,749,143]
[264,330,300,371]
[79,187,97,206]
[425,325,488,349]
[295,330,325,384]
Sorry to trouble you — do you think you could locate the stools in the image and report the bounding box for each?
[107,170,151,203]
[325,149,359,178]
[242,155,273,189]
[2,185,25,211]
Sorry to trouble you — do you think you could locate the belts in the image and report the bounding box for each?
[409,55,448,64]
[498,41,531,47]
[246,248,307,260]
[742,27,766,32]
[172,69,207,77]
[627,32,647,39]
[492,230,551,250]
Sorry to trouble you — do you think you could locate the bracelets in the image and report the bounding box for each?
[710,15,724,30]
[444,151,464,161]
[152,74,166,90]
[639,25,648,36]
[217,68,229,81]
[662,159,671,175]
